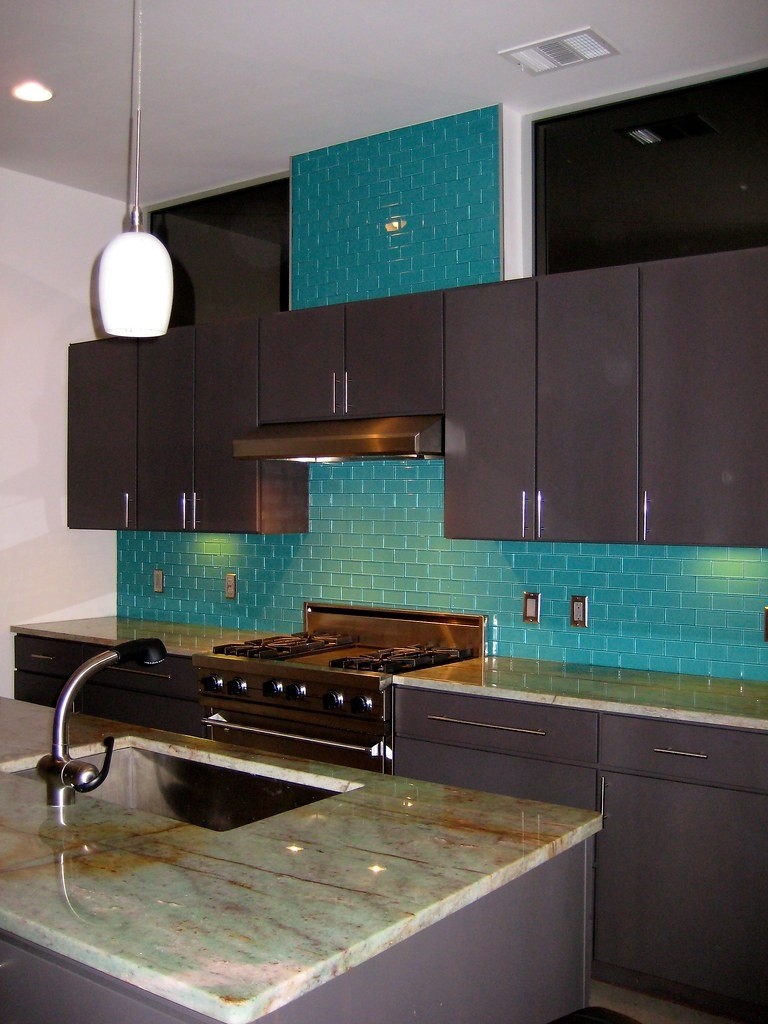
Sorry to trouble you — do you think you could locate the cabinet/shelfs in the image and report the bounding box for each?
[67,249,768,548]
[394,656,768,1024]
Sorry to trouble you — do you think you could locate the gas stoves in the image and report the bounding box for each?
[197,601,488,716]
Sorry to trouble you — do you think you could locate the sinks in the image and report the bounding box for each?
[0,734,368,833]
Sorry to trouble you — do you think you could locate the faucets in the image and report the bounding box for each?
[36,637,170,807]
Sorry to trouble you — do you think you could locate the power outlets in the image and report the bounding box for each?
[153,570,163,592]
[571,596,587,628]
[523,592,540,624]
[225,574,236,599]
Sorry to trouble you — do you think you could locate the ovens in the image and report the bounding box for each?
[202,706,393,780]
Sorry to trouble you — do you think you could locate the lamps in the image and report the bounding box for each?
[98,0,174,338]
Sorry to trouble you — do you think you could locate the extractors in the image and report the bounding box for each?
[232,417,448,461]
